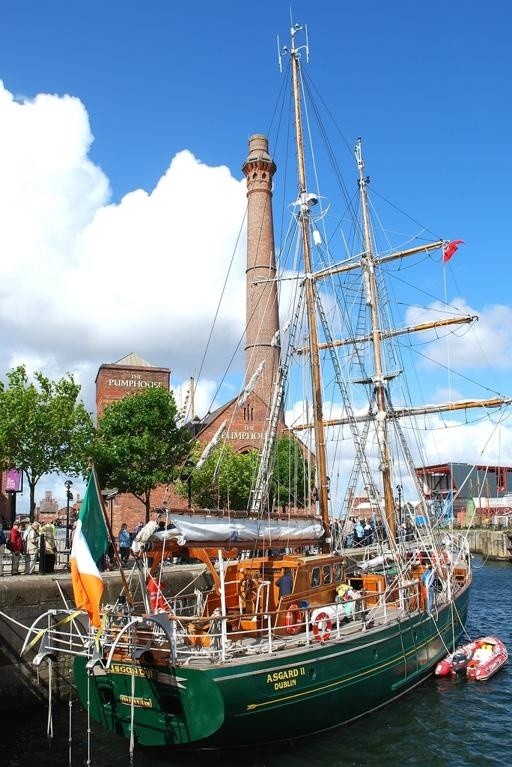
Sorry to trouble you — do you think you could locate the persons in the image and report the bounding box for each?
[146,566,169,642]
[1,514,417,575]
[275,570,293,602]
[421,563,436,611]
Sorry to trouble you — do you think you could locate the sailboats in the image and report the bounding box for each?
[17,1,511,767]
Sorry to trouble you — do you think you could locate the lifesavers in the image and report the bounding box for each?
[285,604,304,634]
[314,613,331,642]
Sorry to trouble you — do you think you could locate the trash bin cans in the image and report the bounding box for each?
[39,554,55,574]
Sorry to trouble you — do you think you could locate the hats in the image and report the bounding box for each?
[11,521,20,526]
[32,521,40,526]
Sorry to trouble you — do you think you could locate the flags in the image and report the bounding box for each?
[70,468,112,629]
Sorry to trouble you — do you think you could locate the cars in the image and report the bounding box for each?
[1,530,40,564]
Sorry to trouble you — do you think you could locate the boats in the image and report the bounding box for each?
[434,634,511,683]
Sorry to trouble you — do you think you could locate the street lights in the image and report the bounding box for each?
[60,478,75,549]
[325,475,332,496]
[395,480,404,526]
[183,458,195,510]
